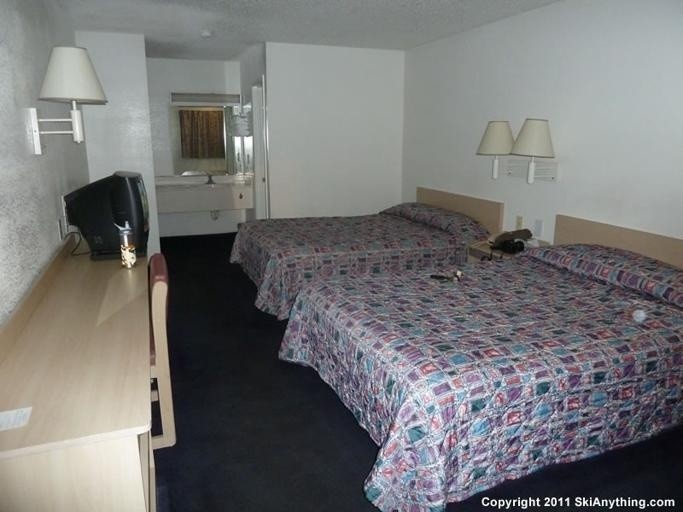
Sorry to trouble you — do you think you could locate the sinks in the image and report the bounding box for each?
[189,184,229,191]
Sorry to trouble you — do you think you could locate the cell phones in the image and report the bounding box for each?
[430,274,447,279]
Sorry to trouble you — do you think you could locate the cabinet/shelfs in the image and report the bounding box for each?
[0,233,178,512]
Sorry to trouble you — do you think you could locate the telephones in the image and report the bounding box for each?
[486,233,499,245]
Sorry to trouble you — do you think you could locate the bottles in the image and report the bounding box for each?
[118,229,137,268]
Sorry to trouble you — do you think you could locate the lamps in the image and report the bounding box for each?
[476,119,555,184]
[29,45,108,156]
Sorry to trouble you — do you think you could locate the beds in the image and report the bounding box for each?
[277,215,683,511]
[229,186,504,320]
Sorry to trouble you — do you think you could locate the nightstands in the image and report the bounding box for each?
[468,239,549,263]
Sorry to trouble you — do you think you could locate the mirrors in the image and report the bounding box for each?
[169,106,226,172]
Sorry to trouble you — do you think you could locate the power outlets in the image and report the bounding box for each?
[58,216,70,240]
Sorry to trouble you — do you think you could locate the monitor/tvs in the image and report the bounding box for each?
[64,170,149,260]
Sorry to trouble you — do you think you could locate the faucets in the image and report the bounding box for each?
[205,173,216,184]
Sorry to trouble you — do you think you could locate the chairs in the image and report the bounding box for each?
[147,252,179,450]
